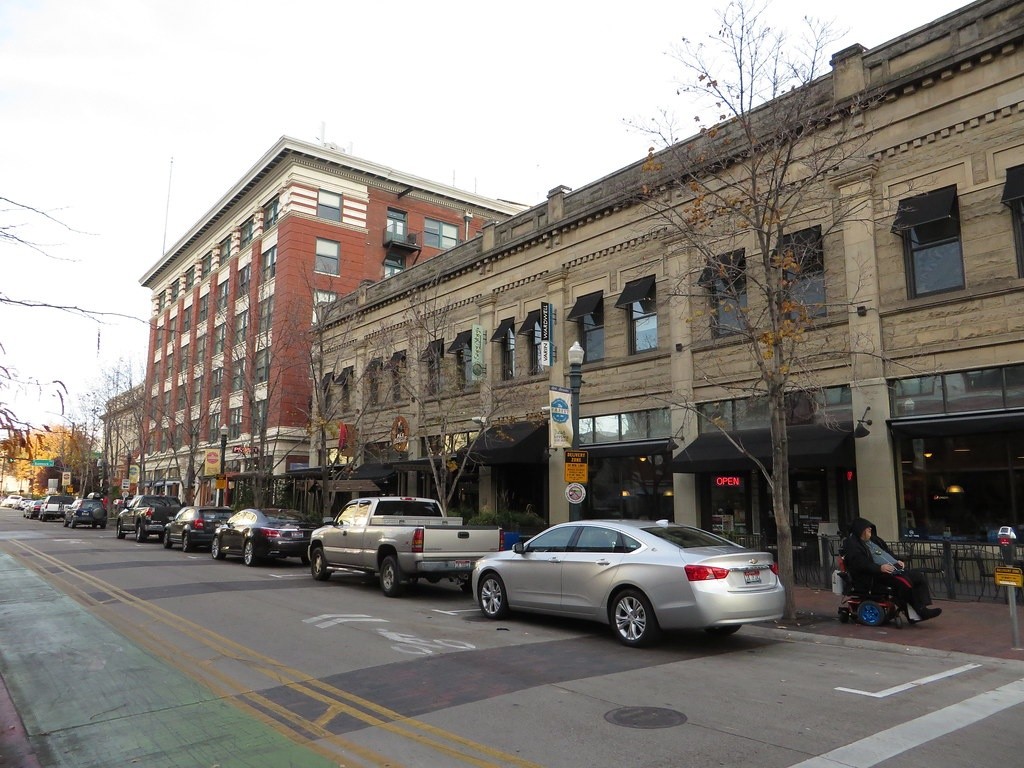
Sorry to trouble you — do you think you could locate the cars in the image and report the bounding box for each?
[23,500,45,518]
[471,518,788,649]
[163,505,236,553]
[211,508,321,567]
[0,495,32,510]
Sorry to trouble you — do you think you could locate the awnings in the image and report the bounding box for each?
[665,421,856,471]
[457,420,549,465]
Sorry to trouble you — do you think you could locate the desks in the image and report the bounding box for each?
[932,545,976,593]
[765,544,806,584]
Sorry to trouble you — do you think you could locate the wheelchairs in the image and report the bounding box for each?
[831,543,923,628]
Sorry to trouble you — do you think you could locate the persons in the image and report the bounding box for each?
[844,516,943,626]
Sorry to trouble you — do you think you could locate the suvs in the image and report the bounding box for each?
[37,495,77,522]
[63,499,107,529]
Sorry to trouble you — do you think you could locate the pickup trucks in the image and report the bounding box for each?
[307,496,508,598]
[115,495,184,544]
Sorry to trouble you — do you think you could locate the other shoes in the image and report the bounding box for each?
[922,608,942,620]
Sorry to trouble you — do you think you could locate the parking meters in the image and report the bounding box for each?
[997,524,1020,650]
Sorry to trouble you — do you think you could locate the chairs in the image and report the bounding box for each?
[590,532,614,547]
[760,537,1024,603]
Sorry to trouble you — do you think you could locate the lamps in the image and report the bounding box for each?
[471,417,487,429]
[951,434,976,452]
[541,406,550,415]
[662,487,674,496]
[946,483,965,493]
[618,487,630,496]
[851,406,873,438]
[639,454,647,461]
[922,448,933,458]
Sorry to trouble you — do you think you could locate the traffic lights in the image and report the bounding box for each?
[8,453,14,463]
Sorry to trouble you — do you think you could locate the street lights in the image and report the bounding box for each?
[218,423,229,508]
[568,341,585,524]
